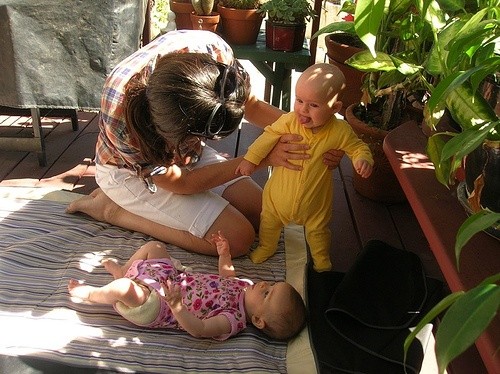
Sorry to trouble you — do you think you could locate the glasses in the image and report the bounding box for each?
[187,60,238,137]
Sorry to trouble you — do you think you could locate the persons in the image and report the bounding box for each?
[67,229,310,343]
[232,62,376,274]
[64,28,344,258]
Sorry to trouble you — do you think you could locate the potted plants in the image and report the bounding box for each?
[310,0,500,374]
[190,0,220,34]
[256,0,318,51]
[170,0,194,30]
[218,0,266,45]
